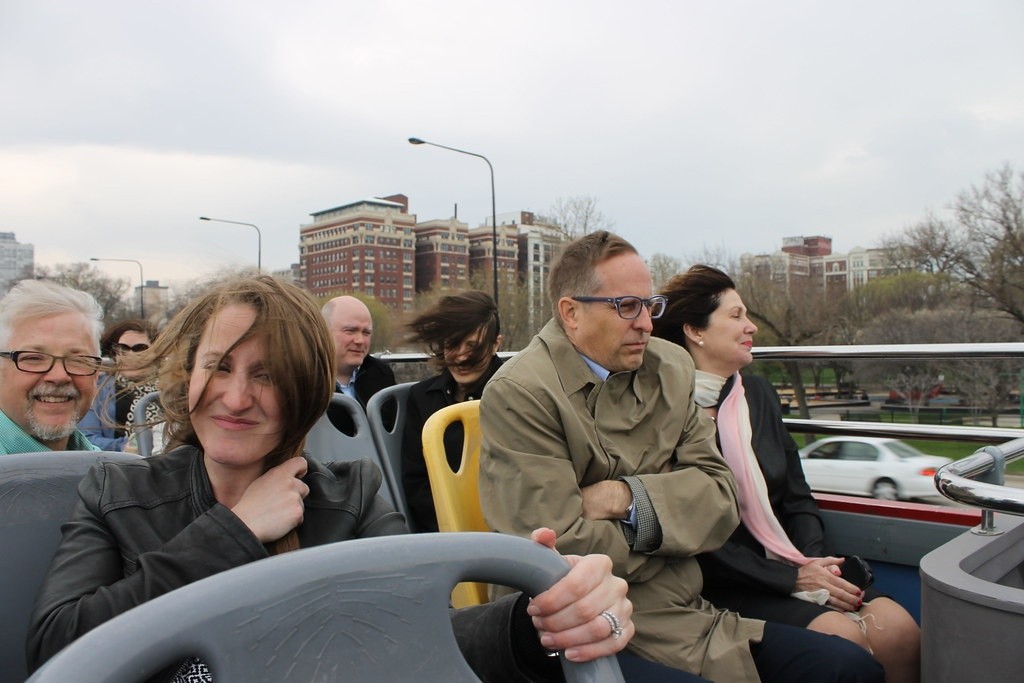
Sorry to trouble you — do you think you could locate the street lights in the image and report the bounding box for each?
[88,257,147,323]
[199,214,265,277]
[407,136,500,308]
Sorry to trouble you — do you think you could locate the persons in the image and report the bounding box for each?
[25,266,636,683]
[76,317,165,454]
[0,278,104,459]
[392,287,505,533]
[321,296,396,436]
[478,229,888,683]
[649,262,923,683]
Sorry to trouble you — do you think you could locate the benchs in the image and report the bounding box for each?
[918,414,963,426]
[838,413,881,422]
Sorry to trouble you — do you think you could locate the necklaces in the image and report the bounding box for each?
[711,418,716,422]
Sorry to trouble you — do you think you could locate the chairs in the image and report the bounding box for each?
[0,451,146,683]
[133,392,395,511]
[366,381,417,531]
[419,398,494,608]
[24,531,627,683]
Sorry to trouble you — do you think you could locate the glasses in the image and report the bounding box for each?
[572,295,669,319]
[0,350,102,375]
[112,343,148,352]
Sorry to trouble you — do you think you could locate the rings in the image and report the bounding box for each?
[599,611,623,640]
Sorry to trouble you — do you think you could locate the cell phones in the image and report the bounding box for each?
[838,555,875,590]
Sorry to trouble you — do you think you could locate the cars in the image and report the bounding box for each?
[797,435,958,503]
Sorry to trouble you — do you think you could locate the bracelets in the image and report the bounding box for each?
[626,494,634,515]
